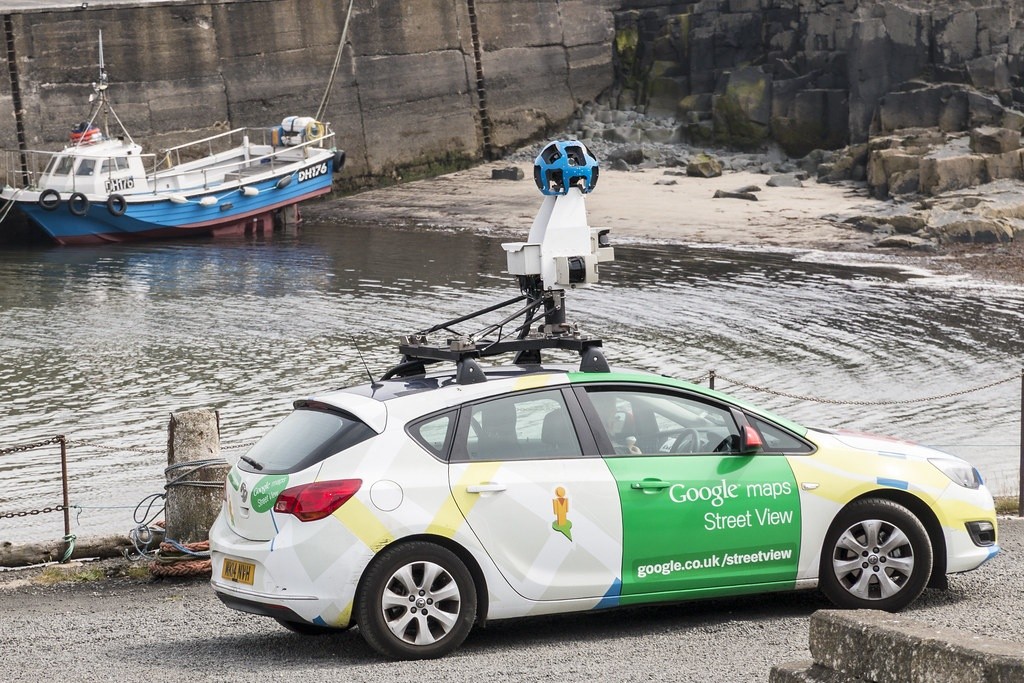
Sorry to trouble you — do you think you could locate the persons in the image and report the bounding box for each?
[591,393,643,454]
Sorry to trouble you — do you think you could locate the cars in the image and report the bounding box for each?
[207,137,1003,661]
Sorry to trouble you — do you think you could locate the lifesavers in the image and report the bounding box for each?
[67,192,90,216]
[38,187,62,210]
[106,193,128,217]
[70,127,101,142]
[333,149,346,173]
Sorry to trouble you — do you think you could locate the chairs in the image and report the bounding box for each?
[478,401,521,460]
[541,407,582,457]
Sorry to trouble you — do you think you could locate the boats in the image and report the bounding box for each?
[0,29,348,248]
